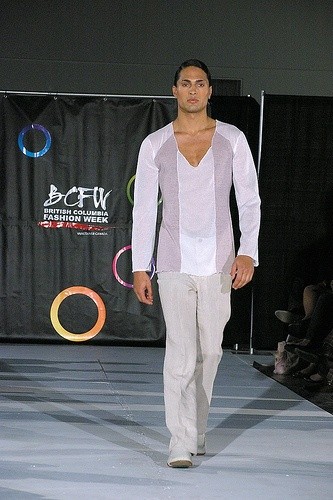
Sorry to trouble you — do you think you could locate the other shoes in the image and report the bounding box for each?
[274,309,303,324]
[167,457,192,468]
[284,339,317,355]
[293,360,318,378]
[191,432,206,455]
[288,317,310,339]
[303,369,329,384]
[297,345,322,363]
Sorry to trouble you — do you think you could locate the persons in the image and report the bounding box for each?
[132,59,261,468]
[275,278,333,383]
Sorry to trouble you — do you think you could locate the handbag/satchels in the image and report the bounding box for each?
[274,341,301,376]
